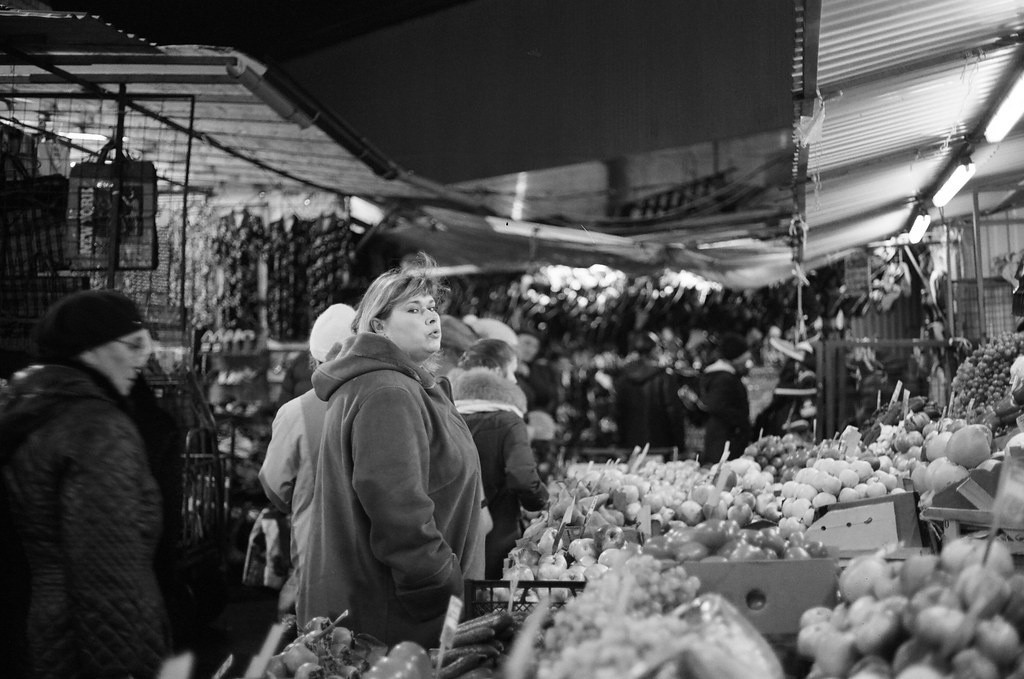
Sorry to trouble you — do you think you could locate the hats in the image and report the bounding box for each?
[44,291,145,360]
[309,303,358,362]
[720,336,749,361]
[440,315,480,351]
[464,314,519,346]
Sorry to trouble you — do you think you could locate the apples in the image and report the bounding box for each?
[488,411,1024,600]
[795,534,1024,679]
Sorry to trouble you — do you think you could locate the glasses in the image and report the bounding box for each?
[115,338,150,363]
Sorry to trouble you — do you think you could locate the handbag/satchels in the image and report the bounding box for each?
[242,507,290,585]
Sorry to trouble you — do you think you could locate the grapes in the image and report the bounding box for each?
[948,331,1024,420]
[535,554,704,678]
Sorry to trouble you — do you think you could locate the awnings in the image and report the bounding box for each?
[0,0,1023,290]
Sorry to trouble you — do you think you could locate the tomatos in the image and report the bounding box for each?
[361,639,432,679]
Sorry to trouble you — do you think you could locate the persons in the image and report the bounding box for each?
[0,293,170,679]
[608,328,766,466]
[256,267,552,653]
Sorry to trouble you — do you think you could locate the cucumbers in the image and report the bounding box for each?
[428,610,528,679]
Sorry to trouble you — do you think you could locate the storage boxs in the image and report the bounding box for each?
[677,488,926,636]
